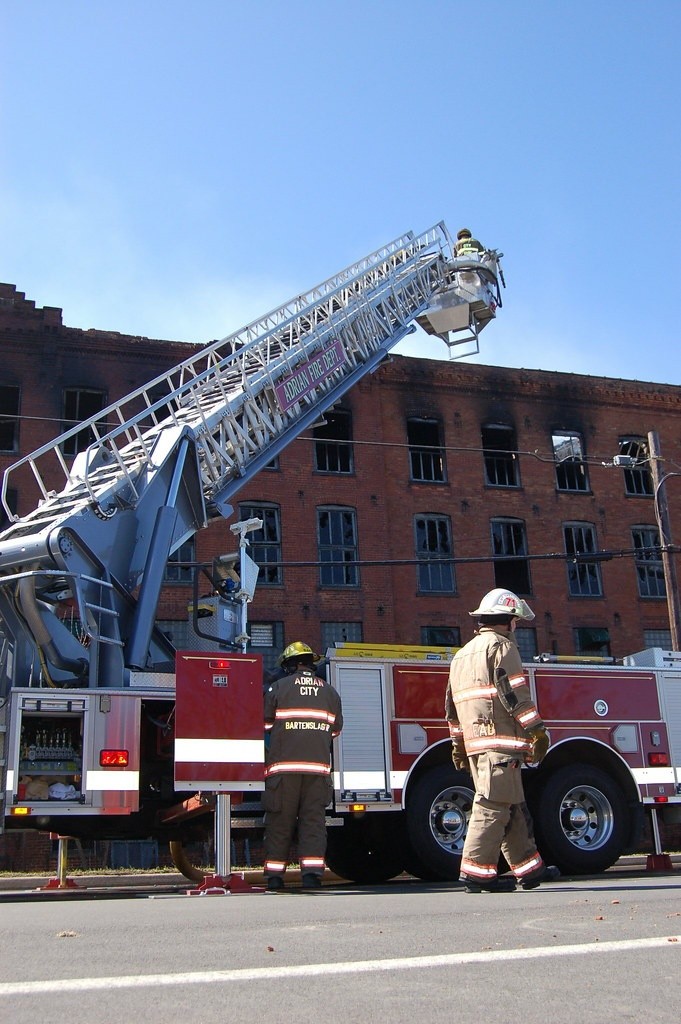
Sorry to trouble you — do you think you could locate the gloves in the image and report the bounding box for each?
[512,701,548,763]
[448,721,468,770]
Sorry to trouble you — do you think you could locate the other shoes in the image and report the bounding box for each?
[303,874,321,887]
[268,877,284,889]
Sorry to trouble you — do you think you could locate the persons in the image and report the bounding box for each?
[452,228,487,267]
[445,587,551,893]
[263,640,344,892]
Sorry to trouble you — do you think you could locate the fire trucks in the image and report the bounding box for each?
[0,218,681,906]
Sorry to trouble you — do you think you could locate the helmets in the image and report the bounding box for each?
[468,589,523,621]
[279,641,320,669]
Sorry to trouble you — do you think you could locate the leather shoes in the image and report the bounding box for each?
[522,866,560,890]
[464,876,517,893]
